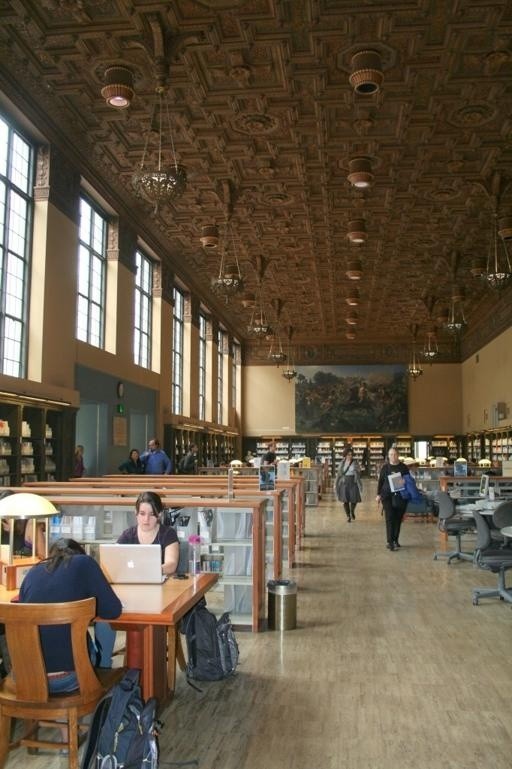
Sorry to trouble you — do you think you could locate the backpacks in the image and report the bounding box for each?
[185,602,239,682]
[80,669,161,769]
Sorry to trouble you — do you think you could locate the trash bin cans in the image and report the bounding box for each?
[267,579,297,630]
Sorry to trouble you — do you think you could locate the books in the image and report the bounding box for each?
[195,507,255,617]
[432,437,512,464]
[0,420,56,487]
[256,440,412,504]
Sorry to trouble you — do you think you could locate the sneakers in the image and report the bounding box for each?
[387,541,400,550]
[347,513,355,521]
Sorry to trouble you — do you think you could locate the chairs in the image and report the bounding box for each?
[374,460,512,606]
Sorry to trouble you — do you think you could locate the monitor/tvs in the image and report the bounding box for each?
[479,474,489,501]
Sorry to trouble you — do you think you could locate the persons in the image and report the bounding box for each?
[295,382,391,419]
[336,447,361,521]
[118,490,181,575]
[140,440,172,476]
[178,443,199,475]
[115,449,144,475]
[207,451,278,474]
[377,448,409,550]
[20,539,122,755]
[1,490,45,560]
[74,445,86,478]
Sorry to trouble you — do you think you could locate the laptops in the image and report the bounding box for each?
[99,544,167,584]
